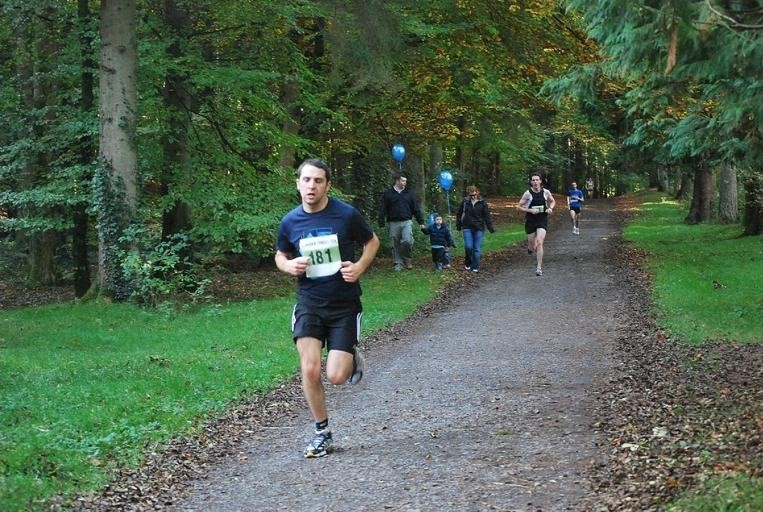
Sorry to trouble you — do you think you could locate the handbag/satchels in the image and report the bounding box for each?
[460,212,465,224]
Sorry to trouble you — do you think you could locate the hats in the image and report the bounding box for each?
[571,183,576,187]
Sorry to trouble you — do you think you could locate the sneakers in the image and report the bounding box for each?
[572,226,579,238]
[347,347,363,385]
[446,264,451,268]
[303,429,335,458]
[536,266,542,277]
[528,245,535,255]
[405,263,412,269]
[464,264,479,272]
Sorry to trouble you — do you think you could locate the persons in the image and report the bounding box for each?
[565,181,585,236]
[272,158,382,460]
[454,185,495,273]
[417,213,457,273]
[584,176,595,201]
[516,170,557,277]
[377,172,424,272]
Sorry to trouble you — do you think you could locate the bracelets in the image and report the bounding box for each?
[549,207,553,210]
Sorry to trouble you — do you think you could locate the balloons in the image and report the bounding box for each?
[435,169,454,193]
[390,143,405,162]
